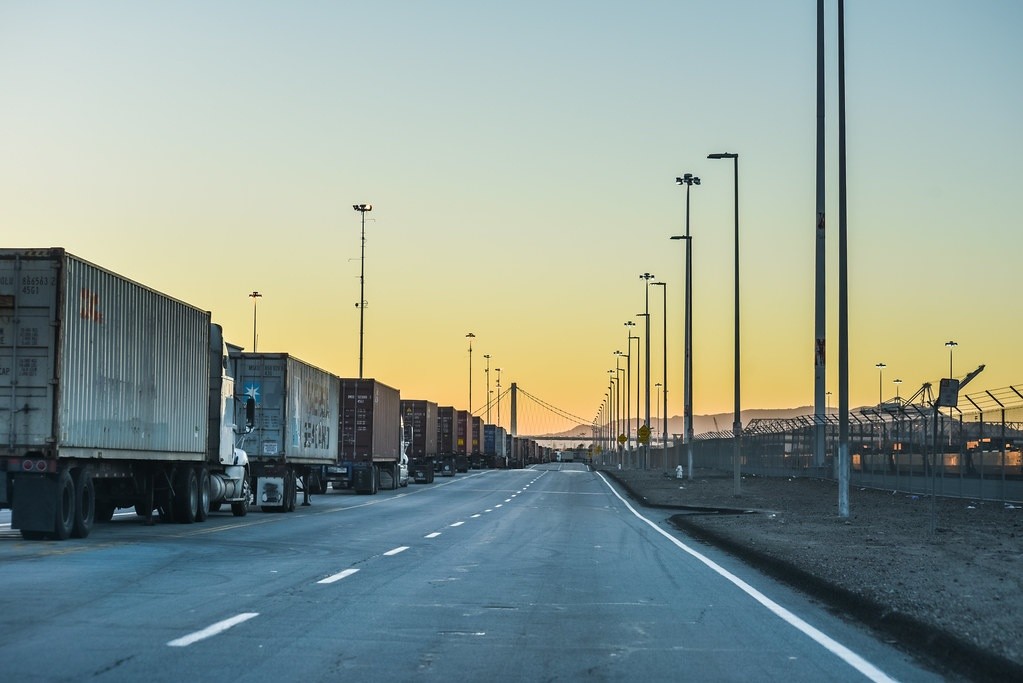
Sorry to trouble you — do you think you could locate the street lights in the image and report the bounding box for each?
[591,354,630,466]
[893,378,903,412]
[249,292,262,353]
[875,362,887,449]
[825,392,832,414]
[676,173,694,445]
[623,321,636,468]
[466,333,475,414]
[671,234,693,479]
[636,313,650,466]
[613,350,622,468]
[654,383,662,443]
[627,336,640,468]
[944,341,958,446]
[650,282,668,477]
[495,368,503,427]
[483,354,492,424]
[639,273,655,471]
[352,204,372,379]
[707,152,743,498]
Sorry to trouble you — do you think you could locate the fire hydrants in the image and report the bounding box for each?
[676,464,684,479]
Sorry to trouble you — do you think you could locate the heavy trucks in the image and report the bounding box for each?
[228,352,576,514]
[0,248,257,541]
